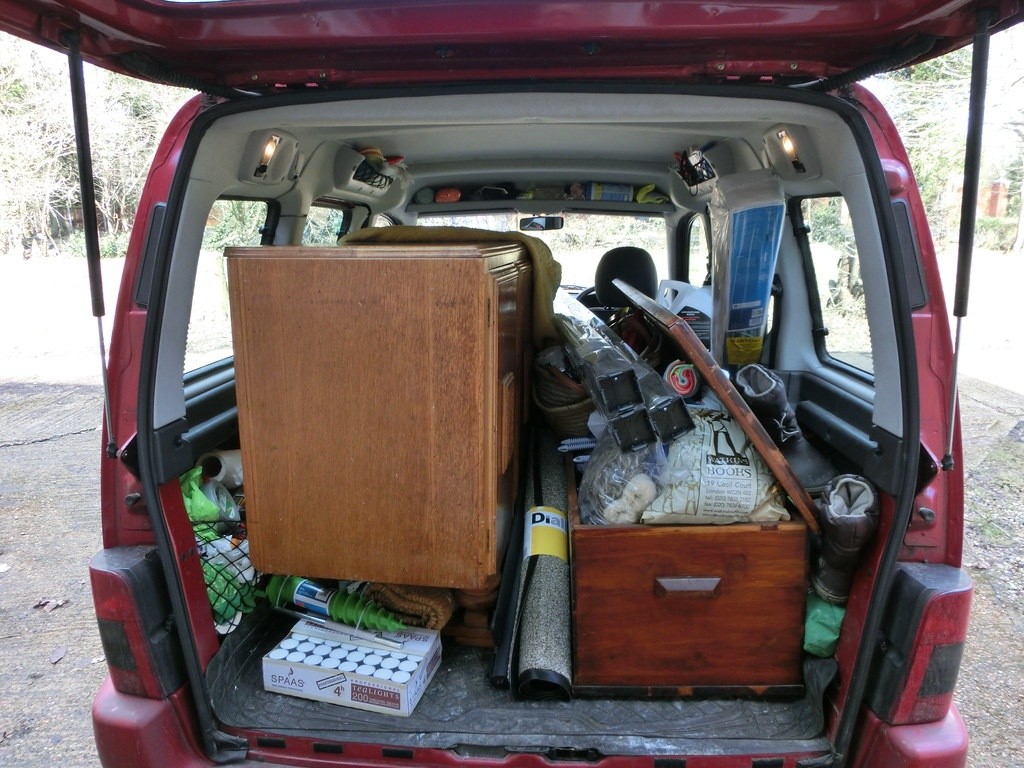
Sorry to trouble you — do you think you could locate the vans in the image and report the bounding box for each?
[0,1,1024,768]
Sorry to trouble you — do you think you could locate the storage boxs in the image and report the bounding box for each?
[261,618,443,718]
[564,279,821,703]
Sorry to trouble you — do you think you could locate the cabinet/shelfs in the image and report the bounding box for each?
[224,241,536,589]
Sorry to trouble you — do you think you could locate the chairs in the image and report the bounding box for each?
[589,247,658,324]
